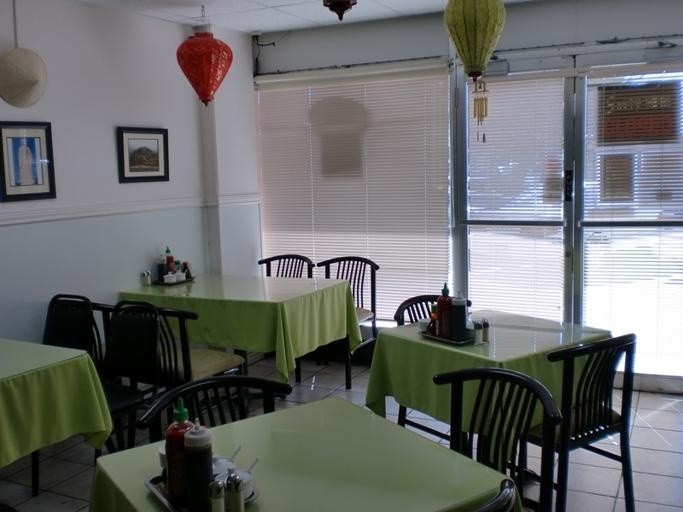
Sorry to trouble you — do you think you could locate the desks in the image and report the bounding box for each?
[0,337,115,512]
[119,274,352,413]
[377,309,612,476]
[95,395,524,512]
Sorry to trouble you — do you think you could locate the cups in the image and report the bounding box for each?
[157,446,165,470]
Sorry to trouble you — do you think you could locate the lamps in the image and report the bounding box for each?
[175,4,233,106]
[442,0,505,143]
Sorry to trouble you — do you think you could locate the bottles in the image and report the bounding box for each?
[184,417,215,483]
[417,281,489,347]
[164,398,194,502]
[206,480,224,512]
[212,455,235,474]
[159,247,189,282]
[216,469,253,499]
[142,270,152,286]
[223,474,245,511]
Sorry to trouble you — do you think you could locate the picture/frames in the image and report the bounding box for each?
[0,121,57,203]
[115,126,169,184]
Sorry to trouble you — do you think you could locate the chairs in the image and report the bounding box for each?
[113,300,245,452]
[431,369,564,510]
[520,333,636,511]
[312,257,379,387]
[48,293,161,452]
[239,254,314,385]
[391,296,472,459]
